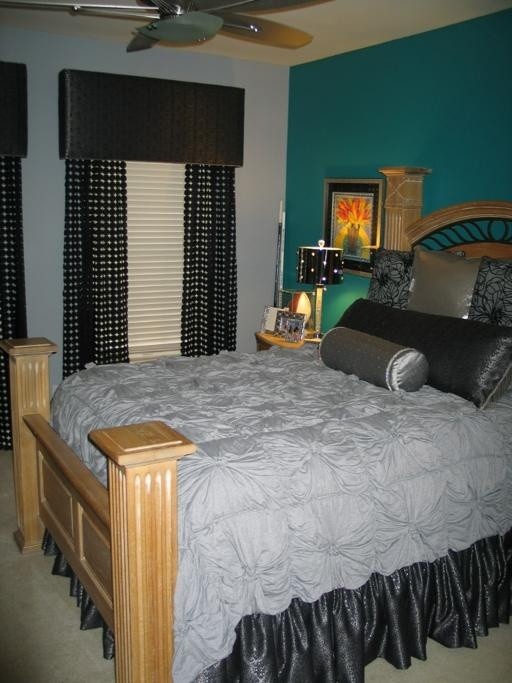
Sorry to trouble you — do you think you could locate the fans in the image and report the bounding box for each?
[126,0,330,55]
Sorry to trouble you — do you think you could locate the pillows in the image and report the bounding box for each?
[319,246,512,408]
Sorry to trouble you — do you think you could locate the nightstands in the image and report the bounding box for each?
[254,329,303,354]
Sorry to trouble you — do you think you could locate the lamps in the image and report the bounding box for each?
[135,11,224,43]
[295,239,344,344]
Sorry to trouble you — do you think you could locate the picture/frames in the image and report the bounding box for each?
[274,309,305,341]
[322,177,387,278]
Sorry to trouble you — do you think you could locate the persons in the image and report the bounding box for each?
[294,326,298,332]
[284,319,288,330]
[288,324,293,333]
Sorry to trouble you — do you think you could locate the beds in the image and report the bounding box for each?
[2,201,511,682]
